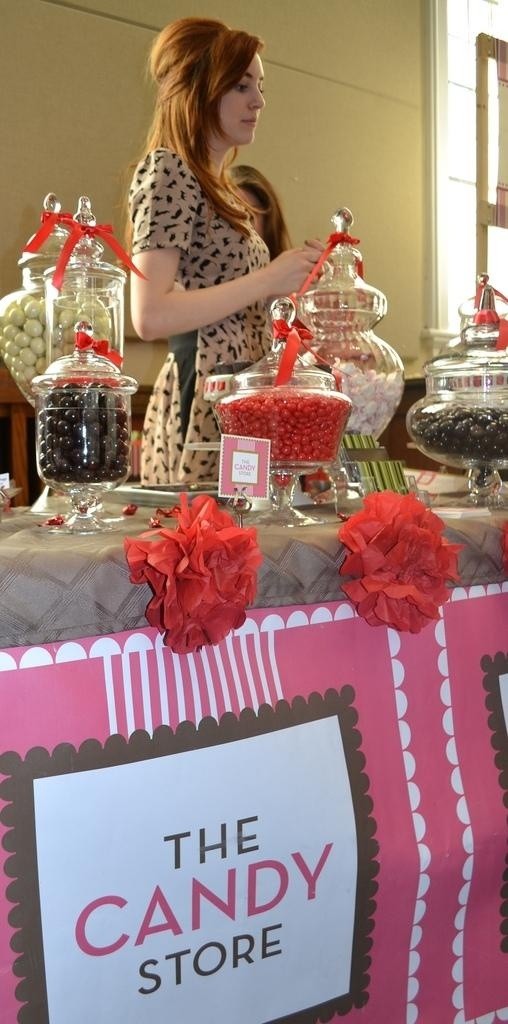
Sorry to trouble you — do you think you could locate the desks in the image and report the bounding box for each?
[0,495,506,1024]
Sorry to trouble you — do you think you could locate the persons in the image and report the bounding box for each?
[216,166,293,281]
[129,16,328,485]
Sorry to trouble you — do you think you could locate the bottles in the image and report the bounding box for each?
[405,288,508,507]
[41,195,128,375]
[0,192,73,403]
[290,208,405,443]
[214,297,352,528]
[31,322,137,535]
